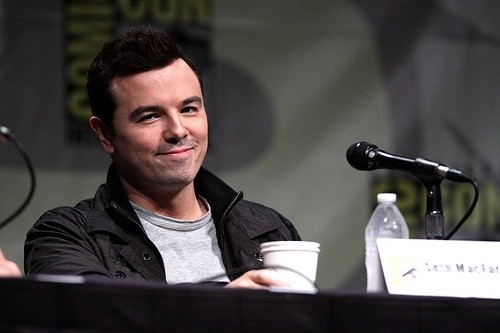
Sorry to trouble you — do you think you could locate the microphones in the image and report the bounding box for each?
[346,142,477,187]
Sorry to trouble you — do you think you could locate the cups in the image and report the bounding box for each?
[258,239,321,294]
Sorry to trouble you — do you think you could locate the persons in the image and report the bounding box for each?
[23,25,320,294]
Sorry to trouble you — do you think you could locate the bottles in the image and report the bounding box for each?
[363,192,411,294]
